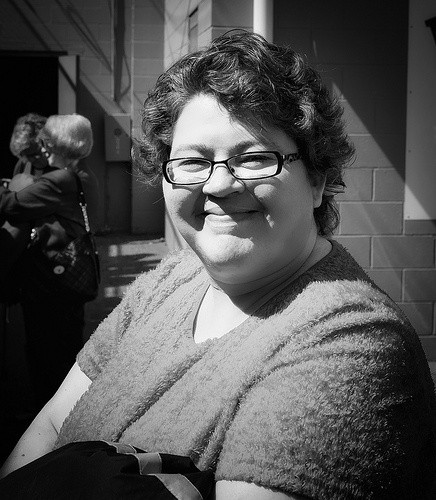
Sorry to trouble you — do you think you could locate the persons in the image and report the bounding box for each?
[0,111,99,412]
[1,29,436,500]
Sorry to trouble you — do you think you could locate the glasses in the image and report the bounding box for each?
[160,151,303,186]
[40,137,52,148]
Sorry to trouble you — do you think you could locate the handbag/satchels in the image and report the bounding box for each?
[14,174,99,301]
